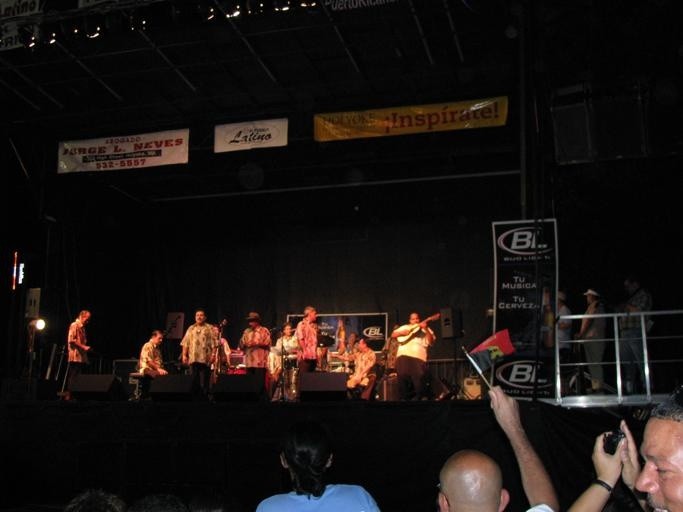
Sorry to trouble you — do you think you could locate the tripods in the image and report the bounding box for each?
[444,353,471,400]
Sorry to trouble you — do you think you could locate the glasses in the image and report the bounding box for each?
[437,483,450,508]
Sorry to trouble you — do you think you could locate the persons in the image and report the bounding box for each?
[565,388,682,512]
[176,473,231,512]
[381,311,436,401]
[607,273,658,397]
[60,308,93,401]
[238,312,271,404]
[544,289,575,395]
[180,310,231,405]
[266,307,376,400]
[255,413,382,512]
[436,384,562,512]
[58,481,127,512]
[136,330,168,405]
[126,473,193,512]
[576,285,611,394]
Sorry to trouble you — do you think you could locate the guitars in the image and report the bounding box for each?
[397,312,441,344]
[210,320,227,365]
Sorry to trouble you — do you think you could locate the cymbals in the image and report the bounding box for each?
[317,336,335,347]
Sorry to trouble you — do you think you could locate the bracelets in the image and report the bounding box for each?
[594,479,613,495]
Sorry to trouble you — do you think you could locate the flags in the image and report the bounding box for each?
[468,329,517,375]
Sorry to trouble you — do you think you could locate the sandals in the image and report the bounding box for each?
[435,387,451,400]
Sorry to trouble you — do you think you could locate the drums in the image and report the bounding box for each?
[284,367,299,401]
[283,354,297,369]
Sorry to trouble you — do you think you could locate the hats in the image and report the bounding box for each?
[584,289,600,297]
[244,312,268,324]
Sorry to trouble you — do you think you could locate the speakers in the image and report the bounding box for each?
[300,371,348,401]
[149,375,194,401]
[24,287,50,321]
[440,307,464,339]
[213,373,273,401]
[112,359,139,400]
[70,374,129,402]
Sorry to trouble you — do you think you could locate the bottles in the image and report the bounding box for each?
[335,319,346,354]
[541,287,555,348]
